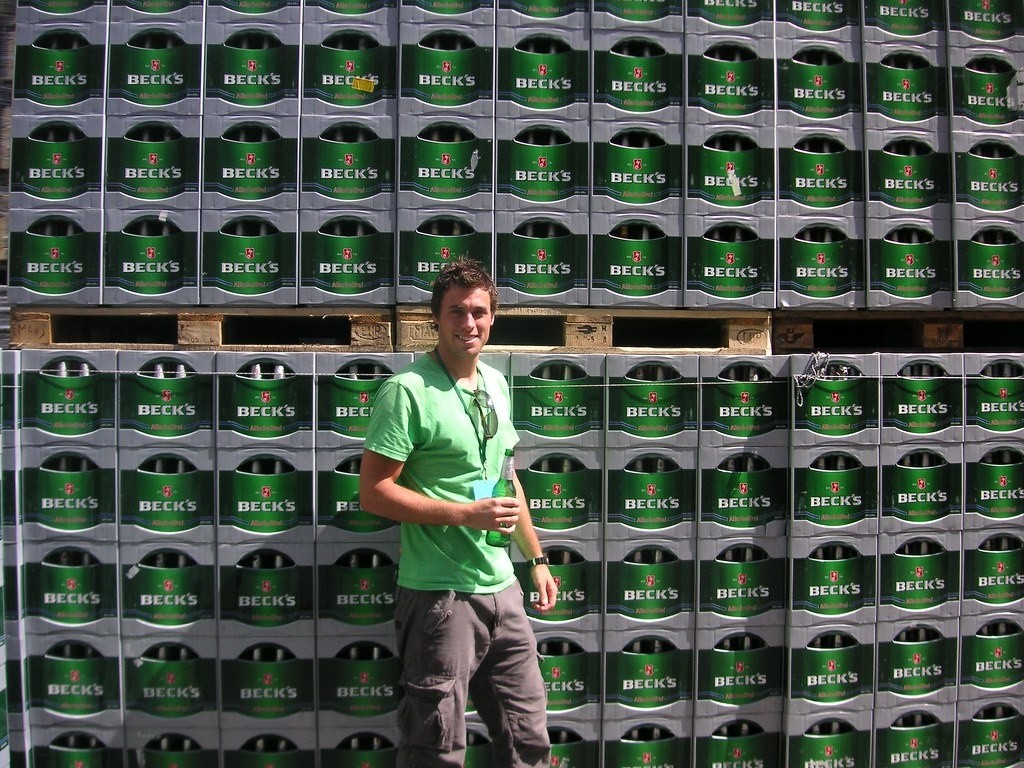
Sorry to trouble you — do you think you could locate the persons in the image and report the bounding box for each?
[360,257,559,768]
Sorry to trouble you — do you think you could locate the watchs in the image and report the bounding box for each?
[525,556,550,569]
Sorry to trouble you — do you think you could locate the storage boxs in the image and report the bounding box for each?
[0,0,1024,768]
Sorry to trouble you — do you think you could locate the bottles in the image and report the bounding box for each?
[485,449,517,547]
